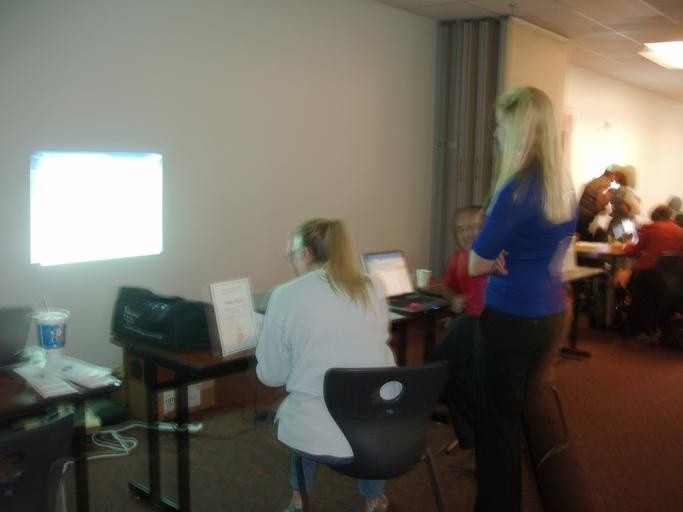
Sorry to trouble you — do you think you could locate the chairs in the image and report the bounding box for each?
[646,252,683,345]
[295,360,448,512]
[0,408,75,512]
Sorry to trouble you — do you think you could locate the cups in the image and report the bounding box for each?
[416,269,432,288]
[36,312,69,362]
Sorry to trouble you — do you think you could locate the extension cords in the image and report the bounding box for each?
[158,421,203,434]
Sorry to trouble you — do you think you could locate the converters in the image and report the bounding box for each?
[254,410,276,420]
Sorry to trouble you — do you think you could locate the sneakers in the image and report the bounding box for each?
[283,496,304,512]
[458,447,479,479]
[365,494,389,512]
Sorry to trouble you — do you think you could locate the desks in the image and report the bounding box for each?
[415,265,609,445]
[0,359,121,512]
[111,289,409,512]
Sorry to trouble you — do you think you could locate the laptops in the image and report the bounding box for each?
[360,250,450,317]
[1,306,34,369]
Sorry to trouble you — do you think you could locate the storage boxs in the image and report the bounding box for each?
[125,377,215,422]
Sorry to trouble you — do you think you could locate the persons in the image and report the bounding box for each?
[576,160,683,354]
[254,216,402,512]
[436,204,490,472]
[466,85,581,512]
